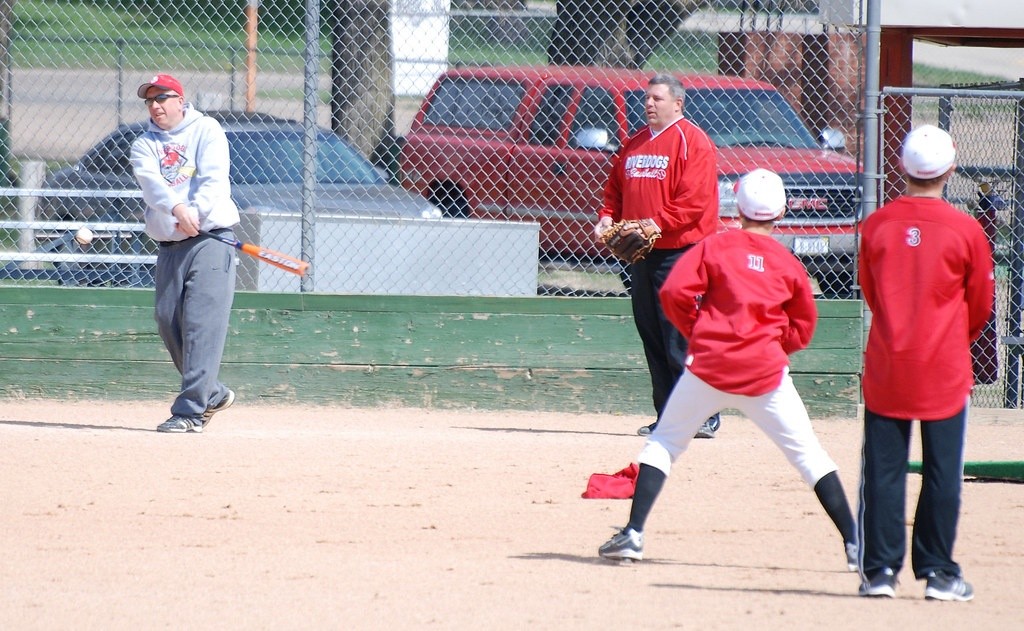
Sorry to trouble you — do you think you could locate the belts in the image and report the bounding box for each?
[160,232,206,247]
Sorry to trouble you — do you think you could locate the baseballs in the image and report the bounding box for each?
[76,227,94,245]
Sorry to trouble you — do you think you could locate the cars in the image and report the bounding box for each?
[36,108,442,287]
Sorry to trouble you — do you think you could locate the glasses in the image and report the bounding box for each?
[144,93,181,104]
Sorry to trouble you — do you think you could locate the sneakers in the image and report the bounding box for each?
[857,569,897,597]
[924,572,973,600]
[158,414,202,432]
[638,421,659,434]
[695,413,719,438]
[598,528,646,560]
[199,391,235,430]
[847,545,863,572]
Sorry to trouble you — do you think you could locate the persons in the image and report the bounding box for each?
[129,73,242,434]
[595,167,863,574]
[594,72,720,437]
[858,124,996,603]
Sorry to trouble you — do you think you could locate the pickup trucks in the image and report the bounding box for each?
[403,66,863,301]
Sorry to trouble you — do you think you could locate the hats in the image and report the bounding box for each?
[136,73,184,99]
[734,168,786,218]
[903,124,953,179]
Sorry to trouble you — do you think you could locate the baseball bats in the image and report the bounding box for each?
[198,230,310,277]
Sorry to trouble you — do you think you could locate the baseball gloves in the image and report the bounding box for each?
[599,217,663,264]
